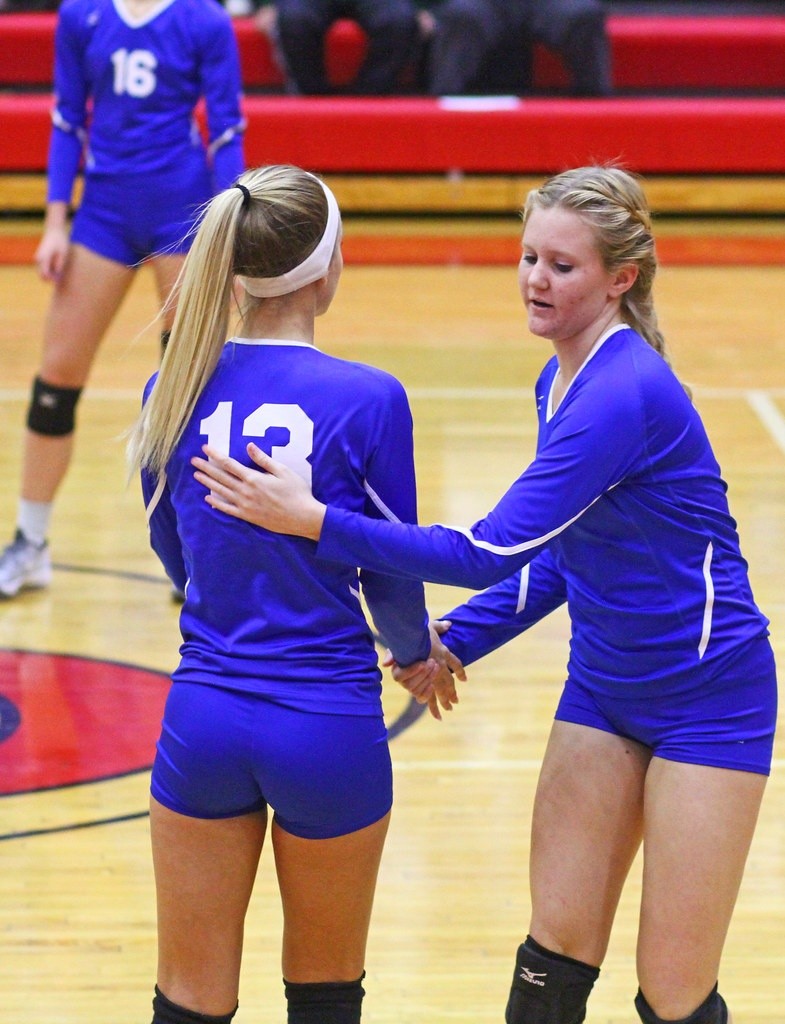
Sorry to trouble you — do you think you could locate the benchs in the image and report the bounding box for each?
[0,9,785,267]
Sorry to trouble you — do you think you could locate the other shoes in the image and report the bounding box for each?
[1,526,53,595]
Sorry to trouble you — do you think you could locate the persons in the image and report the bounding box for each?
[190,166,778,1024]
[224,0,612,98]
[0,0,245,600]
[126,165,469,1024]
